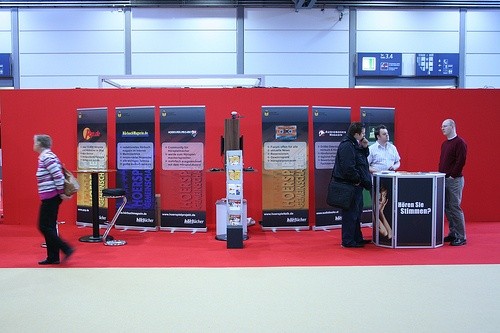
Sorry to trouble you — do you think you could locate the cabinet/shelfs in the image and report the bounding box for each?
[226,150,245,227]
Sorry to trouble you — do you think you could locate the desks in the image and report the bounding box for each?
[372,170,447,248]
[215,198,248,240]
[71,167,118,244]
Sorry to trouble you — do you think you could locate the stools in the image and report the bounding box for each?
[100,188,128,246]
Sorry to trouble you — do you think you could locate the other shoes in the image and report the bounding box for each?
[38,259,60,265]
[62,248,74,263]
[342,239,372,247]
[444,236,456,242]
[450,238,466,246]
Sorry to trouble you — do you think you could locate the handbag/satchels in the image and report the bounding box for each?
[326,180,358,211]
[63,168,79,196]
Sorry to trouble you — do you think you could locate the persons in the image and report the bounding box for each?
[438,119,467,246]
[33,135,75,265]
[368,123,400,173]
[332,122,372,248]
[373,182,392,240]
